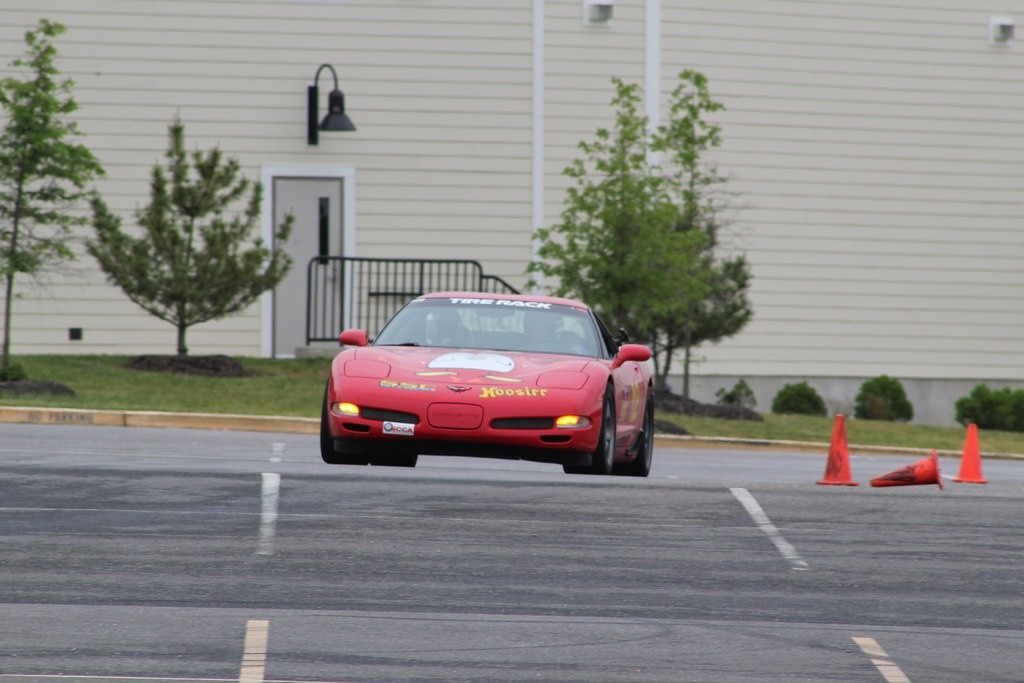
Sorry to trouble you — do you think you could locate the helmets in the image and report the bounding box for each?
[425,307,461,347]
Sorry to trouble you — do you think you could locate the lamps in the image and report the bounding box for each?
[307,64,356,148]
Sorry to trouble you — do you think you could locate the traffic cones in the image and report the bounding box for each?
[951,423,991,483]
[816,413,863,488]
[868,447,946,487]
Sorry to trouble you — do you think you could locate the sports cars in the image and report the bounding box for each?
[316,293,658,477]
